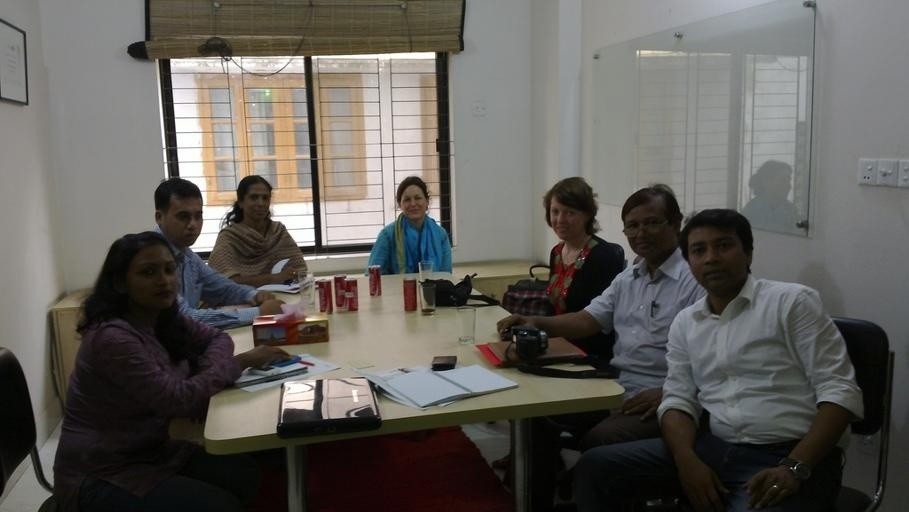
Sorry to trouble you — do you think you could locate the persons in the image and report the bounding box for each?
[571,206,871,512]
[737,156,800,232]
[496,181,709,454]
[490,175,626,471]
[207,174,310,287]
[363,174,455,278]
[48,228,291,512]
[149,175,287,333]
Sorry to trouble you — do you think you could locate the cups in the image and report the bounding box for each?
[416,260,433,281]
[298,269,316,312]
[419,286,436,316]
[456,307,474,346]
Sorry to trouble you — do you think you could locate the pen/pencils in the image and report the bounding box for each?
[299,360,314,366]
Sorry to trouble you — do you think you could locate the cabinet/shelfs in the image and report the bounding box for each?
[450,259,553,308]
[48,291,92,411]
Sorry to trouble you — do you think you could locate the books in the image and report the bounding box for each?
[230,356,310,389]
[382,364,520,408]
[473,335,589,369]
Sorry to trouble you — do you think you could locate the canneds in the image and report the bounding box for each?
[319,280,332,312]
[334,275,346,307]
[344,279,358,311]
[368,265,382,296]
[403,278,417,312]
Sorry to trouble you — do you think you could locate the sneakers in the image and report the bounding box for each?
[491,448,566,489]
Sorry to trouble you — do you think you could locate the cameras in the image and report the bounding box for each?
[508,326,548,357]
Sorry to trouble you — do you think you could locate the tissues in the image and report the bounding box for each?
[251,299,329,346]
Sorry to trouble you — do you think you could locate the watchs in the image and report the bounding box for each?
[776,454,815,483]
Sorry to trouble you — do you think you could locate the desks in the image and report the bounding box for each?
[195,264,629,510]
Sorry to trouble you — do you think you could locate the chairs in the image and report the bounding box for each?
[1,341,56,511]
[825,313,895,512]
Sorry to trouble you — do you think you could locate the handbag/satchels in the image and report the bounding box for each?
[500,278,552,316]
[420,275,473,308]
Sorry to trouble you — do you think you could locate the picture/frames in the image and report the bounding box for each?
[1,17,30,106]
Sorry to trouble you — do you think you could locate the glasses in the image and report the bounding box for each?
[622,218,669,239]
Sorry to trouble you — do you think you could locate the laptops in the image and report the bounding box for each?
[277,377,382,438]
